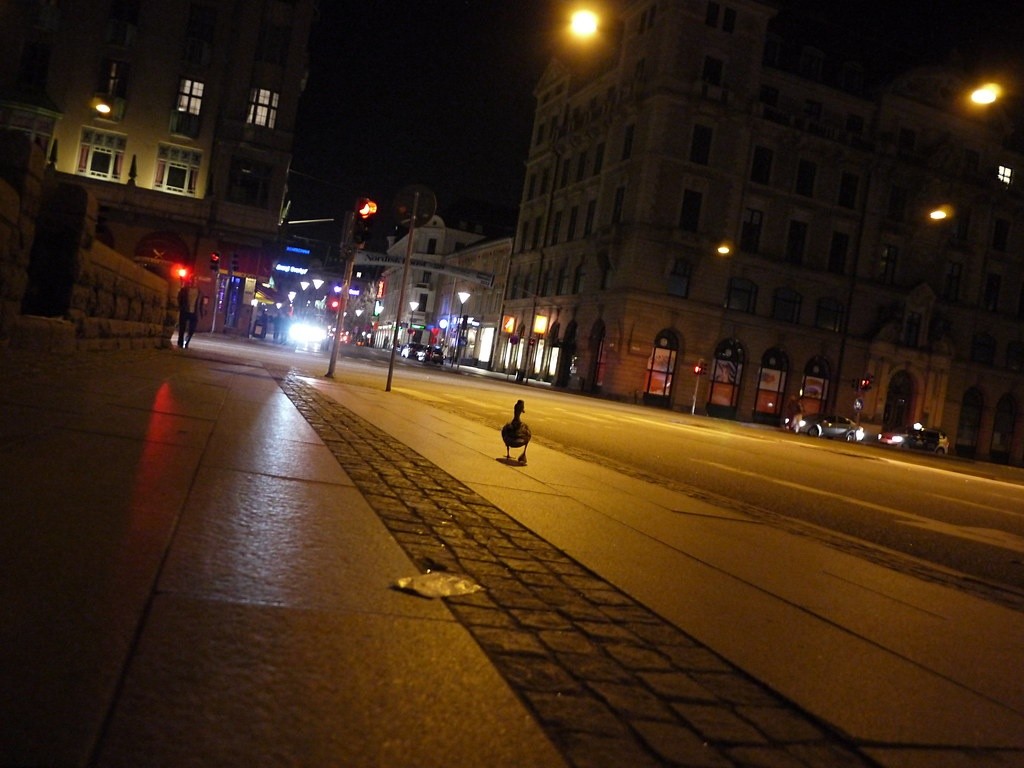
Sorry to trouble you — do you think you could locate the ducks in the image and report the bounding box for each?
[500,399,532,464]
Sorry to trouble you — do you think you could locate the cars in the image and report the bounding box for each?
[785,414,865,443]
[396,343,444,364]
[877,423,950,456]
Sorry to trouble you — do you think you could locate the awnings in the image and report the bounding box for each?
[256,284,284,303]
[134,231,189,268]
[218,243,273,283]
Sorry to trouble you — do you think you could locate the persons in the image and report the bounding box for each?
[442,343,455,364]
[382,336,389,349]
[259,309,268,338]
[271,312,292,344]
[177,273,205,349]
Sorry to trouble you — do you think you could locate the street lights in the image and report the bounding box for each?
[407,301,420,345]
[449,292,470,366]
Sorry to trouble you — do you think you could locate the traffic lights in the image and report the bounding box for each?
[694,366,701,375]
[700,363,708,375]
[230,253,241,273]
[352,193,380,220]
[209,252,220,272]
[374,300,383,317]
[860,379,870,391]
[329,298,341,310]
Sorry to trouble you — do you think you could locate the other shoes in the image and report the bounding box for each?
[185,344,188,349]
